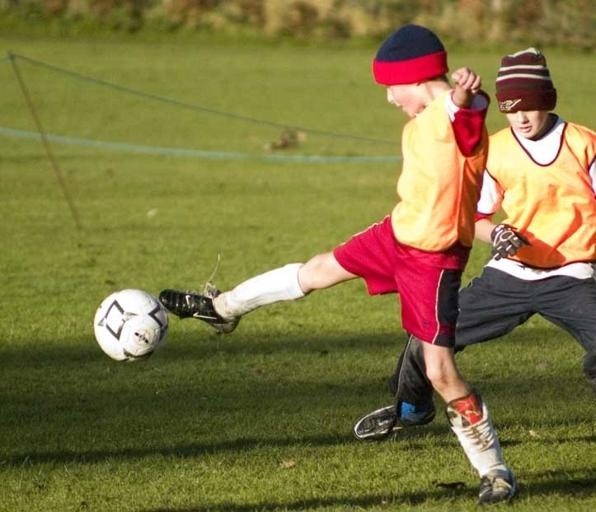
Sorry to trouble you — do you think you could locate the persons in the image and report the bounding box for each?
[351,45,595,442]
[154,21,517,506]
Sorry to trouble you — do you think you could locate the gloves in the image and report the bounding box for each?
[489,223,531,261]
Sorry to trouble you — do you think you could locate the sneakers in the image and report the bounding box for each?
[159,290,241,334]
[475,463,515,505]
[352,401,436,442]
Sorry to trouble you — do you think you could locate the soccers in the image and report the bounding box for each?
[93,289,168,362]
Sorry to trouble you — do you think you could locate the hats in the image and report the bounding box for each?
[495,46,557,112]
[373,23,449,86]
